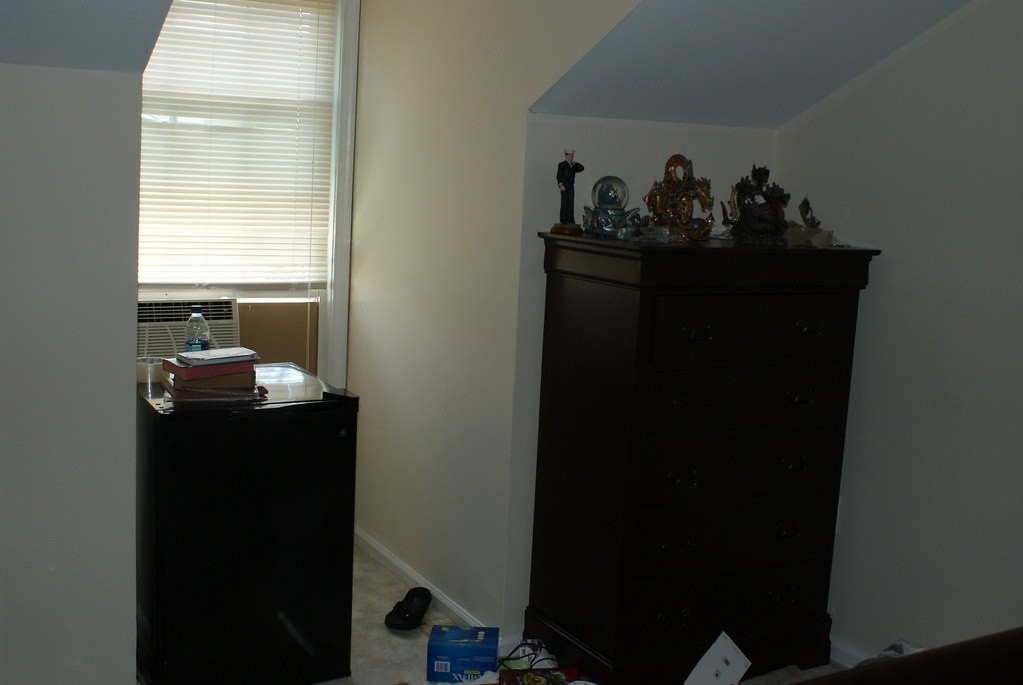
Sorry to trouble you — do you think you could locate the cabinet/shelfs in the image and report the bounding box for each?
[138,360,360,684]
[521,212,885,683]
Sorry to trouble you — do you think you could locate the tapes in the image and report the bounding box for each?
[137,357,170,383]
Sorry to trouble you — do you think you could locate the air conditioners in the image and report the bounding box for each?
[136,297,241,361]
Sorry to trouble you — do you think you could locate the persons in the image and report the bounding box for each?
[556,149,585,224]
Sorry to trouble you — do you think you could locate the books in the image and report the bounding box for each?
[162,346,269,407]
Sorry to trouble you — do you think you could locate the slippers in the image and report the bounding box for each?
[384,586,431,630]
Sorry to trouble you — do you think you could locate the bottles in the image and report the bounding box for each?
[186,307,209,351]
